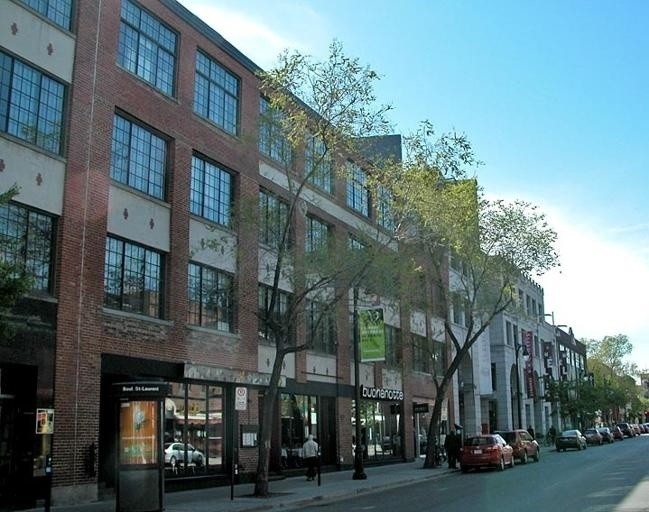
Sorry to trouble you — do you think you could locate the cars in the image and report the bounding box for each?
[492,429,541,465]
[163,441,203,468]
[457,432,516,473]
[582,422,648,446]
[554,428,587,452]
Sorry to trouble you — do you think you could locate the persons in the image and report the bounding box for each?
[527,424,534,440]
[444,429,459,469]
[549,424,557,445]
[303,434,320,482]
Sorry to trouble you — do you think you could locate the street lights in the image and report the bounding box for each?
[555,324,570,432]
[350,271,378,480]
[514,343,529,428]
[535,312,552,437]
